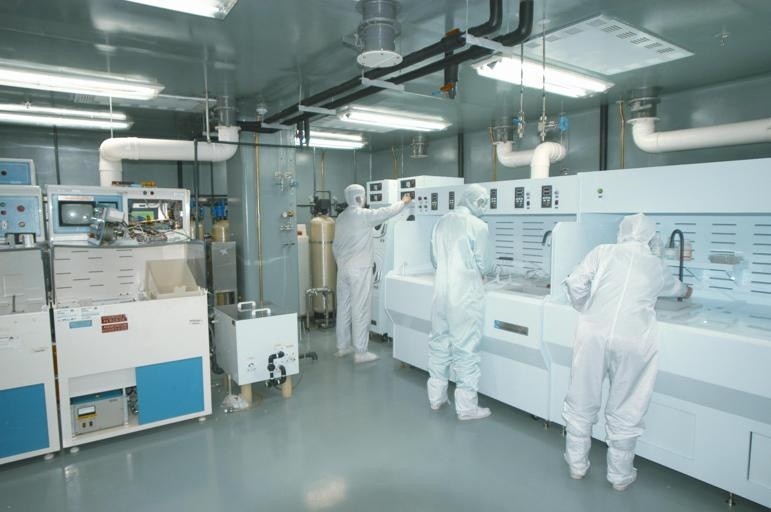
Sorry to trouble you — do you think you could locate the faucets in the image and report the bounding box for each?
[492,263,501,282]
[541,230,552,244]
[668,229,685,300]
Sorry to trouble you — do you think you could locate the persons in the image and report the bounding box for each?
[332,183,412,363]
[426,184,495,420]
[563,212,693,492]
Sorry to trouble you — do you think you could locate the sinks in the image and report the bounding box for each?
[505,283,549,295]
[656,296,703,320]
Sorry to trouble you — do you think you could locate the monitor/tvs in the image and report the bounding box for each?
[59,201,96,226]
